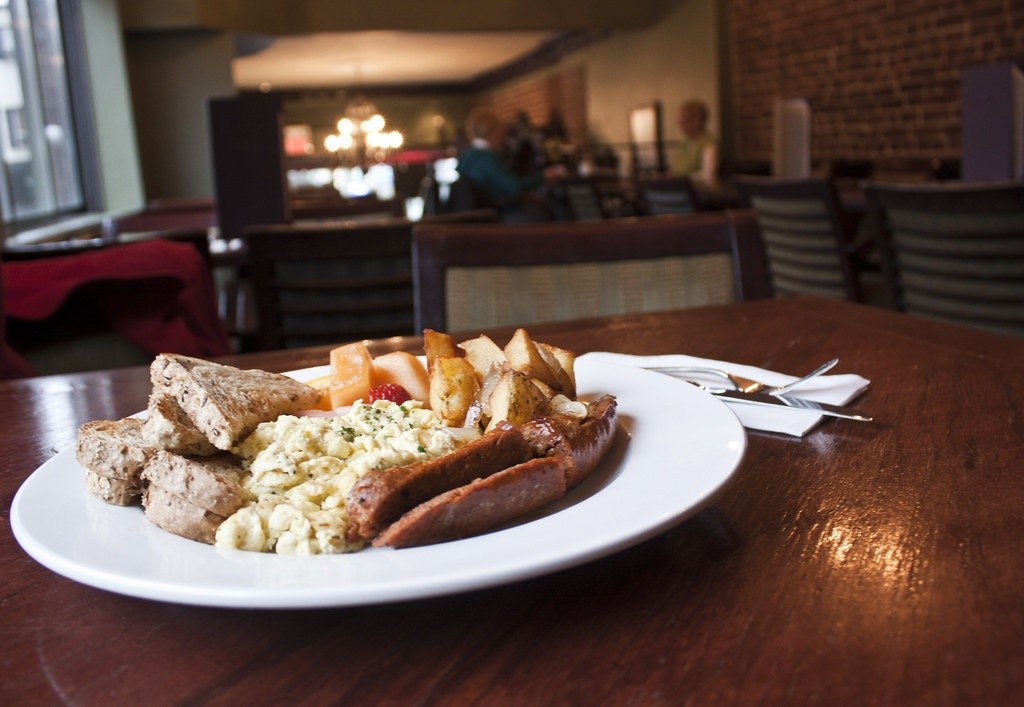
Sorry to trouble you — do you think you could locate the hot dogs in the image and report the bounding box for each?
[334,395,632,546]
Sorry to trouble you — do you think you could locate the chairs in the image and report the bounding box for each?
[728,172,868,305]
[860,177,1024,338]
[556,176,608,221]
[244,208,505,354]
[636,174,704,215]
[411,211,745,339]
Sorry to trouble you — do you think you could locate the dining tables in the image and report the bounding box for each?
[0,294,1023,706]
[523,177,929,273]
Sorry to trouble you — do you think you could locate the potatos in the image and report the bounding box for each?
[328,325,578,434]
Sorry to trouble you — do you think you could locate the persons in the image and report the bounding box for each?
[459,113,565,222]
[668,100,724,191]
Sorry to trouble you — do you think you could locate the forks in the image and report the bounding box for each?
[640,357,841,398]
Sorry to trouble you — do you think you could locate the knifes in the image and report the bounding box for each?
[684,377,881,421]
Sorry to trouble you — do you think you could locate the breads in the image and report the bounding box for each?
[75,351,325,543]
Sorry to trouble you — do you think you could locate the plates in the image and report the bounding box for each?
[11,355,747,609]
